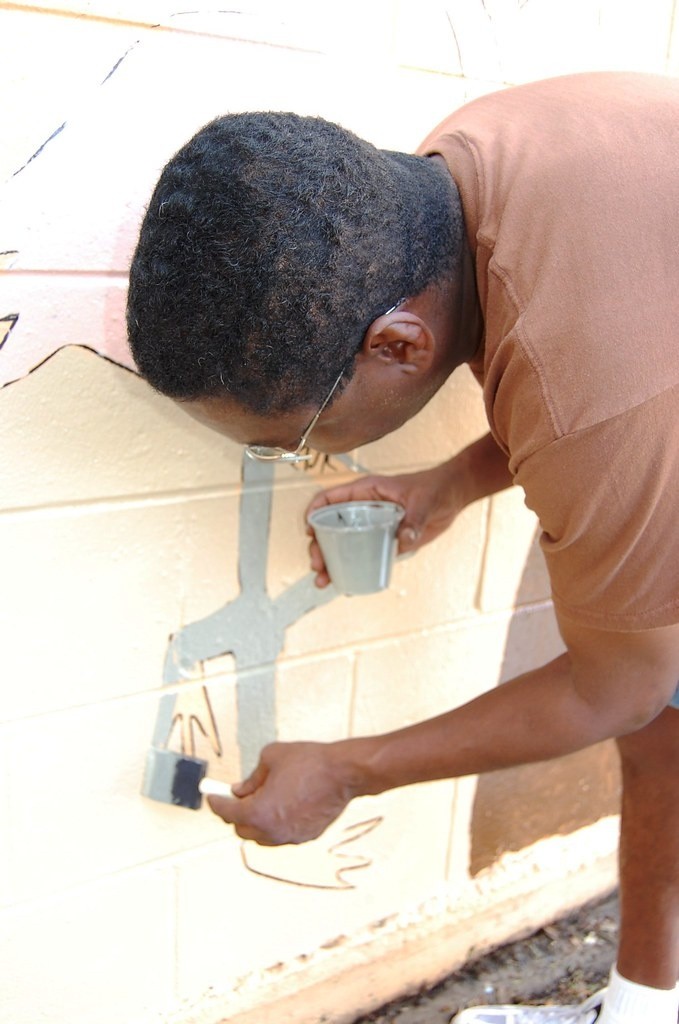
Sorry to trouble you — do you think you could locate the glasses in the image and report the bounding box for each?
[246,296,407,464]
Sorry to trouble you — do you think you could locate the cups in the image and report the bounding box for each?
[306,498,405,597]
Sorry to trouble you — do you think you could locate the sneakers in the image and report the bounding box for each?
[449,987,607,1024]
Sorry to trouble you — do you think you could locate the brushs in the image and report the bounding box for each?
[171,761,240,812]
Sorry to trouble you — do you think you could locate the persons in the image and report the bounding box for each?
[126,69,679,1024]
